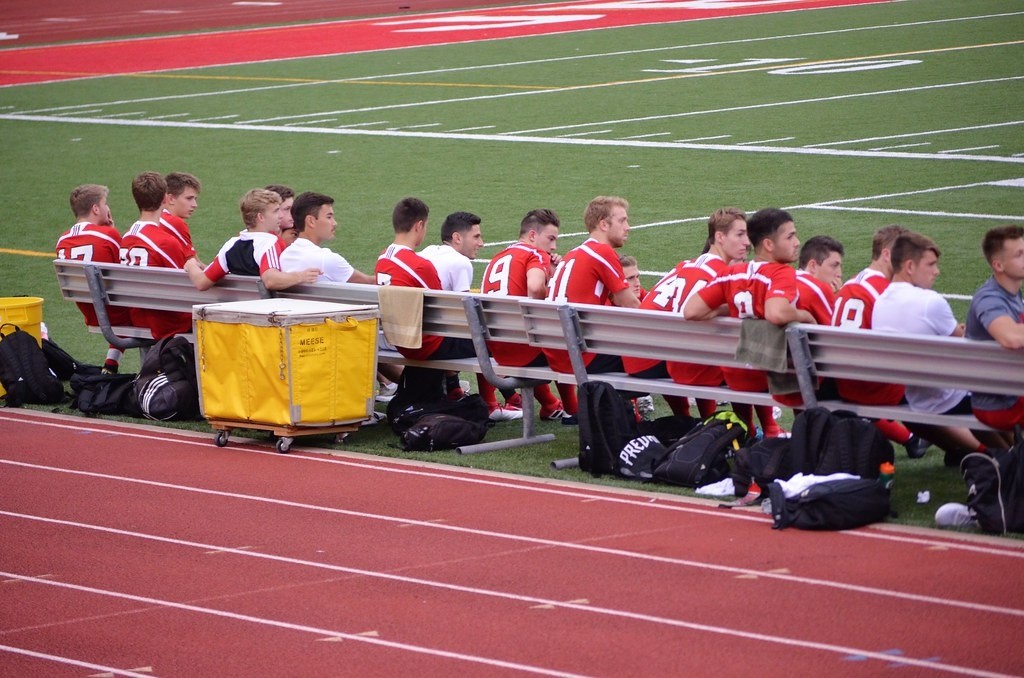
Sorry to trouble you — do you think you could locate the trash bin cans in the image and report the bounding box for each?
[0,296,45,397]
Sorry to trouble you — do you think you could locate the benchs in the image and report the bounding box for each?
[46,261,1024,470]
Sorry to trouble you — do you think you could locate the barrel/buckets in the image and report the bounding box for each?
[0,296,44,397]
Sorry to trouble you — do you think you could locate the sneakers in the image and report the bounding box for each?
[380,382,398,396]
[101,368,120,375]
[489,393,579,425]
[764,429,792,438]
[905,434,932,458]
[935,502,979,527]
[755,427,764,439]
[448,392,478,406]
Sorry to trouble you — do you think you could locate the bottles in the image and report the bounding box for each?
[878,461,895,489]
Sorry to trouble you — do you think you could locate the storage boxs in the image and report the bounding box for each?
[192,298,379,426]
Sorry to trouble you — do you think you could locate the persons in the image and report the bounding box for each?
[55,184,132,375]
[120,171,198,343]
[182,184,1015,469]
[158,172,209,271]
[965,225,1024,431]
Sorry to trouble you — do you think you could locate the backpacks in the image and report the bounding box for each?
[960,423,1024,535]
[400,394,488,452]
[577,381,895,530]
[0,323,206,422]
[386,365,454,436]
